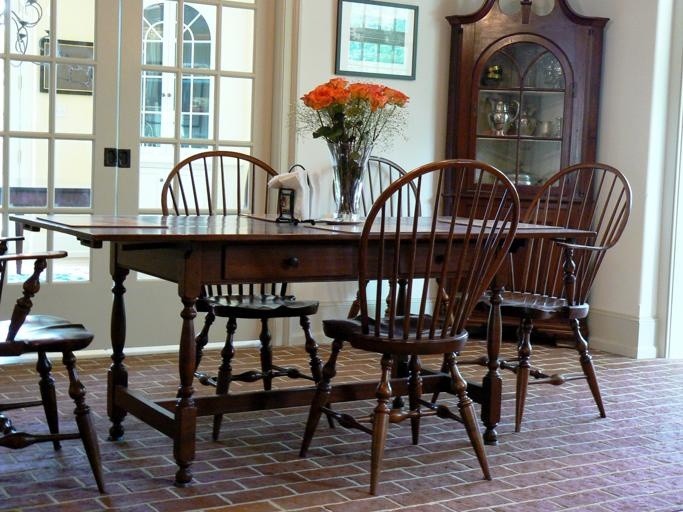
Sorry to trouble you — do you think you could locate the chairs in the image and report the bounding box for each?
[299,158,520,498]
[0,237,105,494]
[332,157,425,220]
[430,162,631,432]
[161,150,335,444]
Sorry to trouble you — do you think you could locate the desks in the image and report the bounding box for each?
[9,215,596,489]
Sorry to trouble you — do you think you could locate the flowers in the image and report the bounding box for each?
[302,79,409,215]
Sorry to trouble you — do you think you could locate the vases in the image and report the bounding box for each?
[327,143,377,222]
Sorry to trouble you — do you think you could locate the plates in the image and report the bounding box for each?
[497,173,532,186]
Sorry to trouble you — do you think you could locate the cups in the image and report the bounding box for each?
[539,120,553,139]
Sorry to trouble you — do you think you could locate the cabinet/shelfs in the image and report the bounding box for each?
[443,0,611,347]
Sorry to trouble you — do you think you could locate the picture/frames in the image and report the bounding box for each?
[39,40,95,95]
[334,0,419,81]
